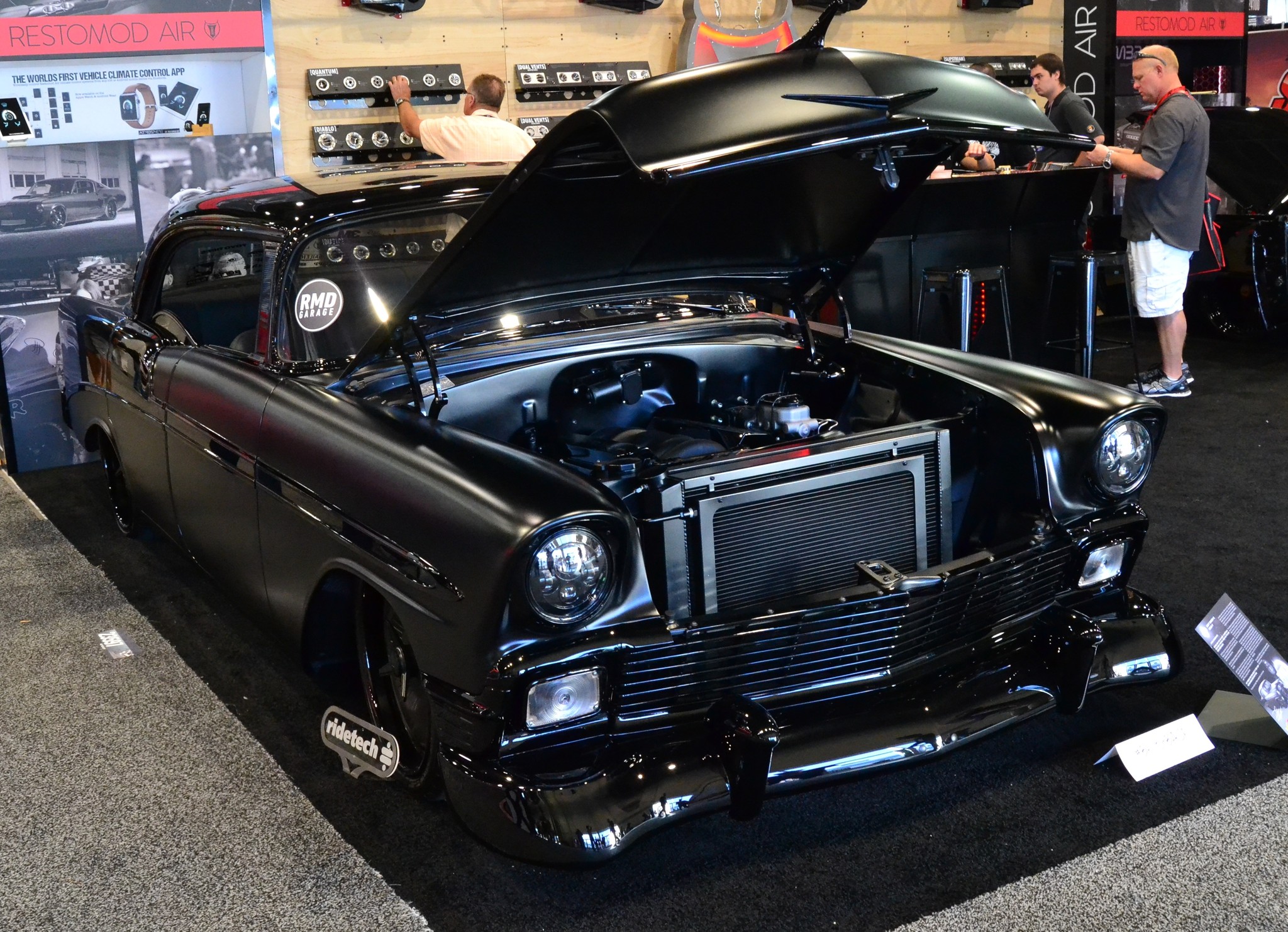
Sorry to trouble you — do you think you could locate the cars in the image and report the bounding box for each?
[0,179,126,231]
[58,46,1181,863]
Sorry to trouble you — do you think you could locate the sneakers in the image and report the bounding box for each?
[1124,373,1191,398]
[1134,362,1194,384]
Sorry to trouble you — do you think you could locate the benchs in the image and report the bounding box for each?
[161,254,435,362]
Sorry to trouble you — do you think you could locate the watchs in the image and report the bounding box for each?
[1102,149,1115,170]
[396,97,411,108]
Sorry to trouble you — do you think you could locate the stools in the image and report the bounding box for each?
[1035,247,1144,395]
[912,260,1016,363]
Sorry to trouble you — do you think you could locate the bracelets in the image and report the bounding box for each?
[974,153,985,161]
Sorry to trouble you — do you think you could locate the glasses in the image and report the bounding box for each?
[1132,51,1167,66]
[463,89,474,96]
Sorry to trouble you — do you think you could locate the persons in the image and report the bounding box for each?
[387,72,538,249]
[939,140,996,174]
[136,155,160,193]
[1030,52,1105,163]
[1087,44,1210,398]
[185,136,219,190]
[959,60,1037,169]
